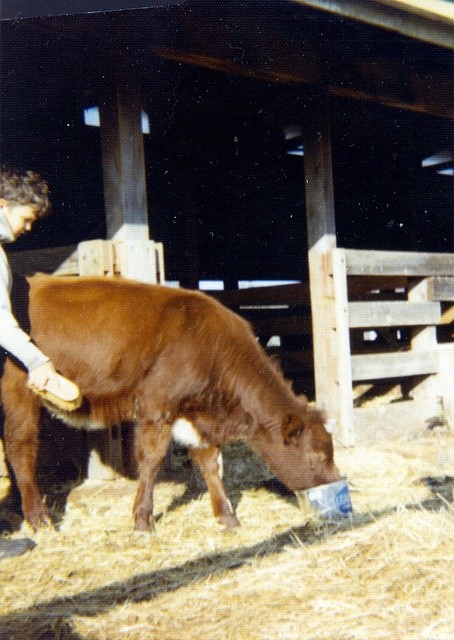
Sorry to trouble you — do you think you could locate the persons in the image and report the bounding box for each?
[0,164,55,391]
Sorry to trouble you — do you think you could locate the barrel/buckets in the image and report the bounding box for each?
[292,476,355,538]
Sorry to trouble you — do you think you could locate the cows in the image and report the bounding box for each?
[0,275,341,541]
